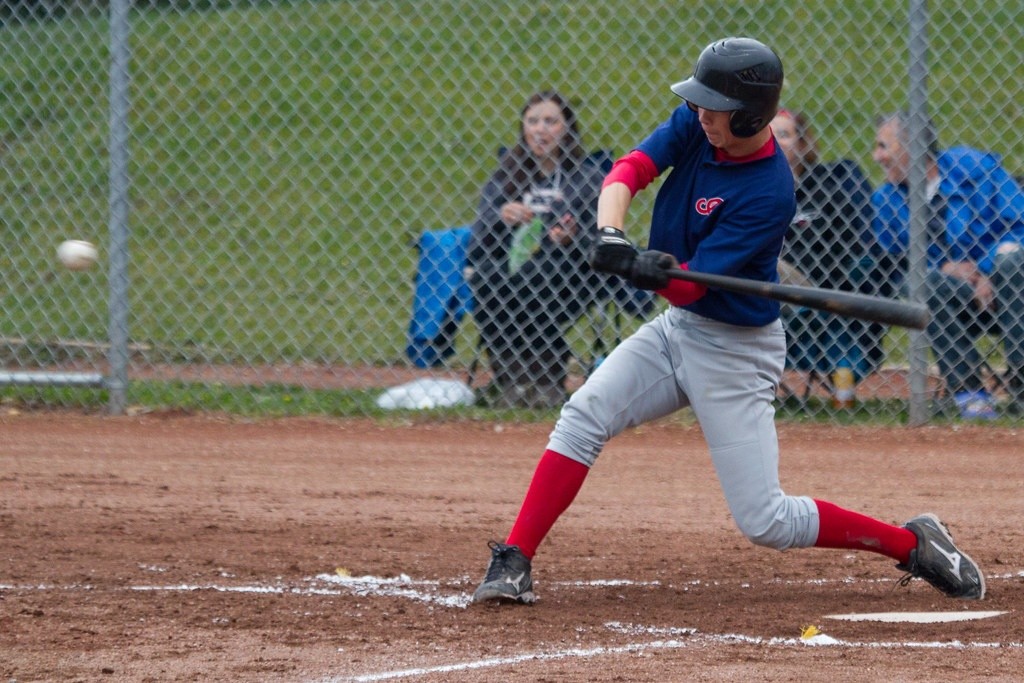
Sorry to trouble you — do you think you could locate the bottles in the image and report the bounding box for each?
[833,360,856,426]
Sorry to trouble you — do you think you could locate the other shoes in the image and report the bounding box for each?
[493,381,568,409]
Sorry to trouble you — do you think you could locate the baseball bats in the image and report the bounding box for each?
[662,264,931,331]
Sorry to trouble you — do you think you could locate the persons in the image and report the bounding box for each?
[748,106,891,418]
[463,90,615,416]
[867,109,1024,420]
[471,35,992,608]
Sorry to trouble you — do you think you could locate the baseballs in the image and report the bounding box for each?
[57,239,98,274]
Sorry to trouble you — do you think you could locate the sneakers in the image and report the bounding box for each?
[949,388,999,423]
[474,544,536,606]
[896,512,986,600]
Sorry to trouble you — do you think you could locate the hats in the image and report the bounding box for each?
[671,37,783,137]
